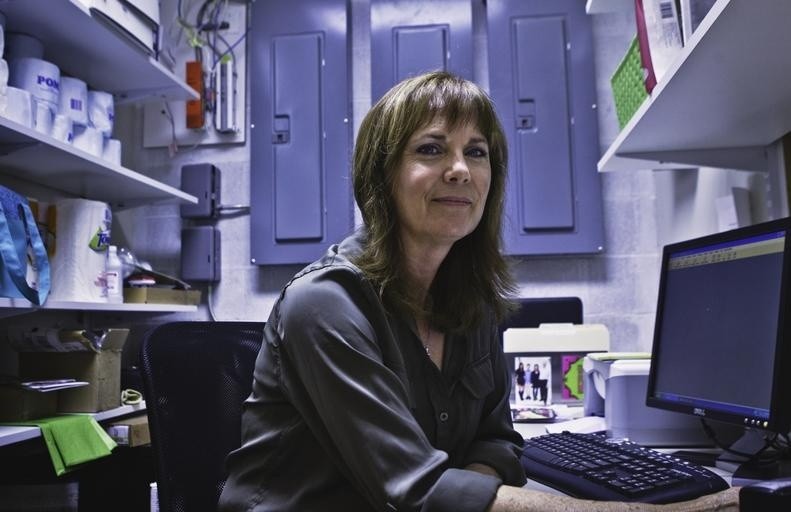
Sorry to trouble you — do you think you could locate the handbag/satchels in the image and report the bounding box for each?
[1,185,51,306]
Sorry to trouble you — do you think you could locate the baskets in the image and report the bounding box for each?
[610,34,647,128]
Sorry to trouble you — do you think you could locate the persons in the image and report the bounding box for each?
[514,362,526,400]
[212,66,748,511]
[523,363,532,399]
[530,364,540,400]
[537,362,549,405]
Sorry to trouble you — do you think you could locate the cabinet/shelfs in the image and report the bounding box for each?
[582,0,791,176]
[0,2,208,445]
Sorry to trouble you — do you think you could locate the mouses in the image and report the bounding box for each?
[738,475,791,511]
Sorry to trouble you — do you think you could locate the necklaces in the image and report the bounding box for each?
[421,322,433,359]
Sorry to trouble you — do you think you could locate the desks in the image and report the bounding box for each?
[505,409,778,504]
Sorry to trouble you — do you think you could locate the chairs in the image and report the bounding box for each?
[138,316,281,512]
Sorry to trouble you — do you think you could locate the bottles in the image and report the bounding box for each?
[107,245,124,304]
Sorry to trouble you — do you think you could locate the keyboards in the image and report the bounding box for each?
[520,429,732,505]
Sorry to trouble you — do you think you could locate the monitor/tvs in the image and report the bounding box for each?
[644,215,791,470]
